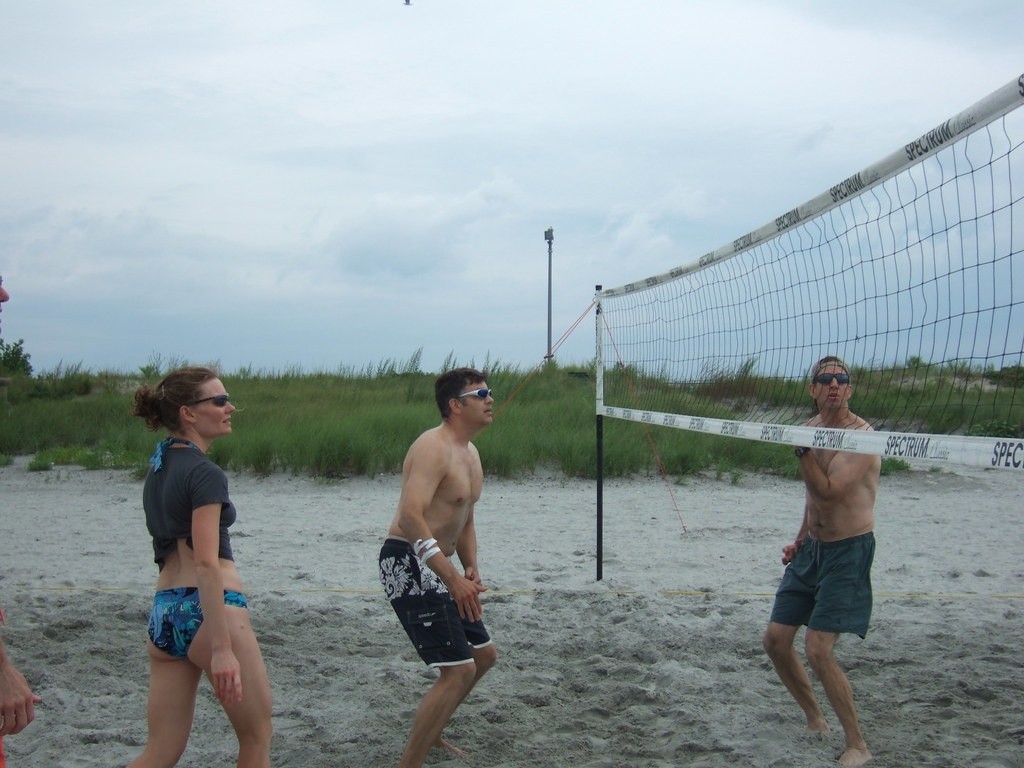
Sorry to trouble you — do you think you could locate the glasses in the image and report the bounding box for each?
[178,395,229,416]
[814,373,850,384]
[454,389,492,398]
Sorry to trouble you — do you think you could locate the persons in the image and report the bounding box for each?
[0,609,42,768]
[763,357,880,767]
[124,367,273,768]
[377,368,499,767]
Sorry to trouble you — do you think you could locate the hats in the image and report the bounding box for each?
[811,356,850,382]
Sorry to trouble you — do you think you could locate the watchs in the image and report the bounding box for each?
[795,447,810,458]
